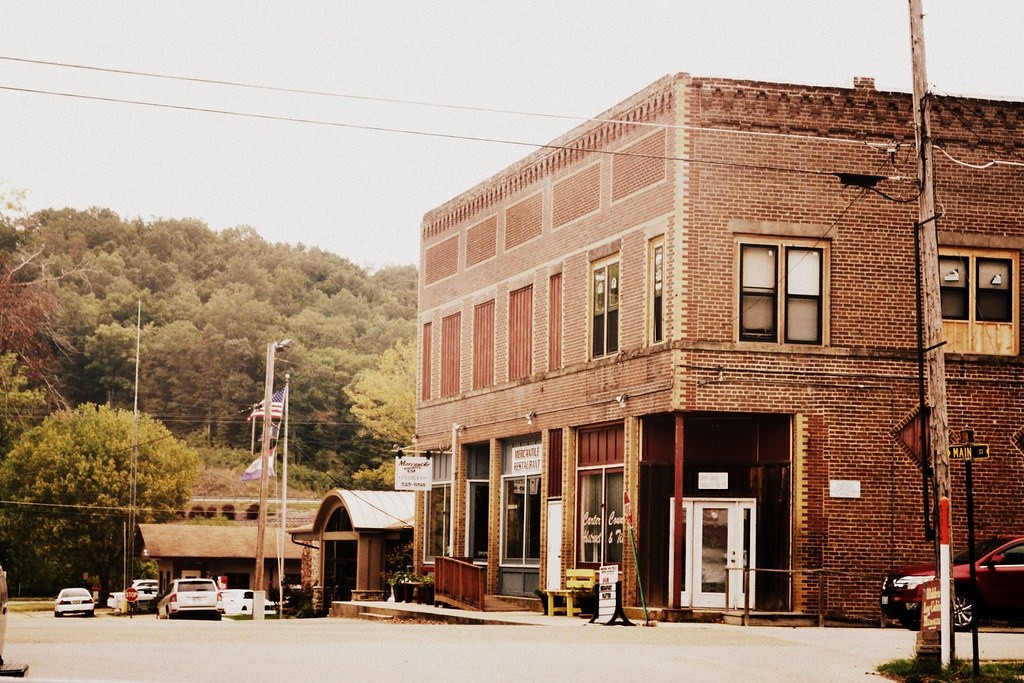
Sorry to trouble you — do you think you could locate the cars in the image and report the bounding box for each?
[106,579,160,612]
[878,533,1024,633]
[54,587,97,617]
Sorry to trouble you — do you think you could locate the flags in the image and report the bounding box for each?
[241,448,276,481]
[258,424,278,441]
[247,389,284,421]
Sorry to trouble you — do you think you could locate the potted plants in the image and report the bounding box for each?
[387,571,435,605]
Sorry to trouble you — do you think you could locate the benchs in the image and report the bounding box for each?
[545,569,597,618]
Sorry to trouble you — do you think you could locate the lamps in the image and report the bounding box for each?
[616,393,629,407]
[411,434,419,443]
[526,410,537,424]
[453,425,467,436]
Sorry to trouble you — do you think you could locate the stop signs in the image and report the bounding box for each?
[124,587,138,601]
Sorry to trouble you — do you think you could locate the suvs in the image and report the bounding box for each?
[157,577,224,620]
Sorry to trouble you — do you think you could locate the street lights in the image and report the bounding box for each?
[253,338,296,620]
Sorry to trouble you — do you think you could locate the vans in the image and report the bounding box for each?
[220,589,277,618]
